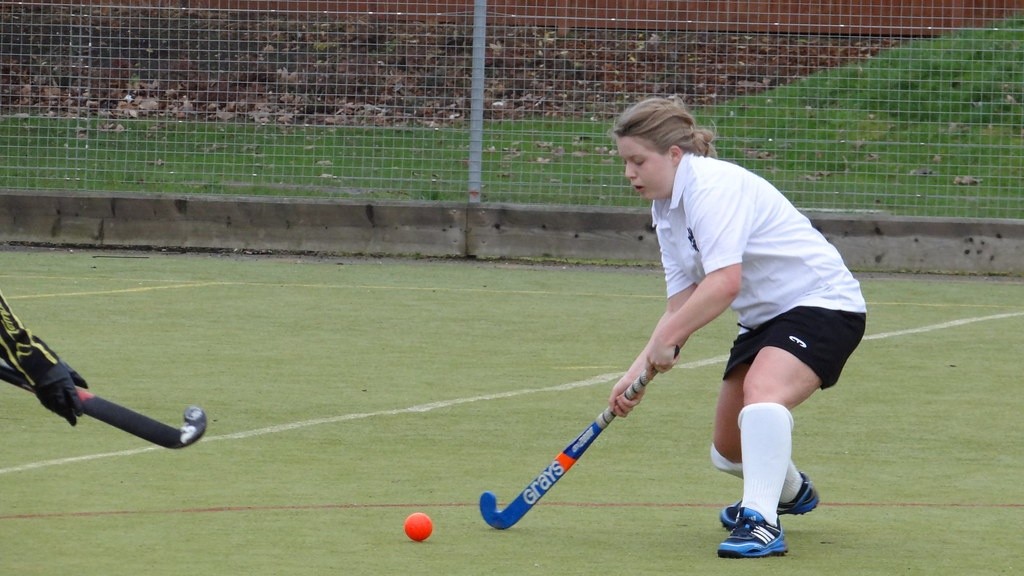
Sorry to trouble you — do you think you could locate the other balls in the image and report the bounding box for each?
[404,513,433,542]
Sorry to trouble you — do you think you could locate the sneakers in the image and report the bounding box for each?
[720,470,820,531]
[717,508,789,558]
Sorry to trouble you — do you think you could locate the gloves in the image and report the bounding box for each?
[36,357,89,428]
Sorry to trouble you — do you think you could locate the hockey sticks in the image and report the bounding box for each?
[480,345,681,530]
[1,363,207,450]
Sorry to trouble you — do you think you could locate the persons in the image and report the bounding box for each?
[605,96,867,564]
[0,292,88,428]
